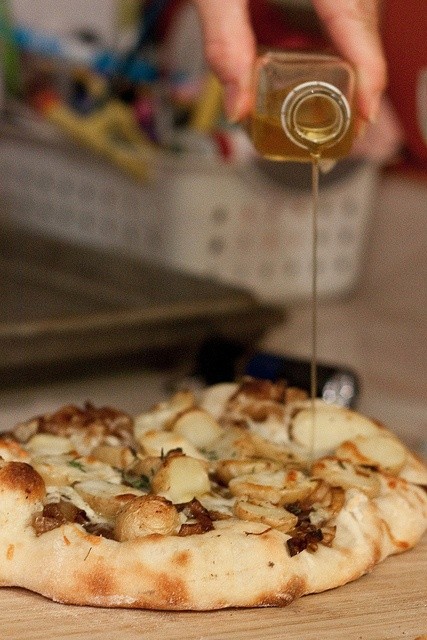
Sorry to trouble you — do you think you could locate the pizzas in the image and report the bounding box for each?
[2,374,426,613]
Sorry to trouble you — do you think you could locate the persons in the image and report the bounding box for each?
[196,0,387,142]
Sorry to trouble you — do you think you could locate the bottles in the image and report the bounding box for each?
[252,49,360,167]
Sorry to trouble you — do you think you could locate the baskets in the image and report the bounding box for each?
[0,112,377,304]
